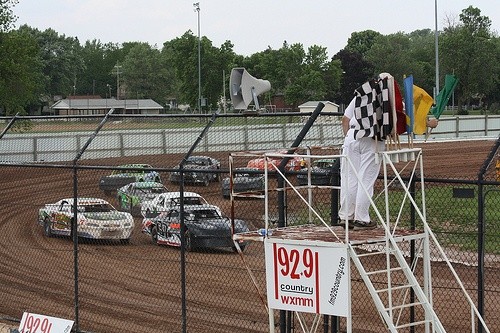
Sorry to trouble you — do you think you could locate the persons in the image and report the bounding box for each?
[338,72,438,231]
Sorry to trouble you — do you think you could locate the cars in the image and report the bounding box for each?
[113,181,170,215]
[167,155,221,186]
[219,166,269,197]
[37,197,133,241]
[297,156,342,185]
[140,191,207,222]
[247,148,310,174]
[99,163,161,194]
[141,205,250,252]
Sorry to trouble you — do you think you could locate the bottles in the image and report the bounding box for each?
[256,229,273,236]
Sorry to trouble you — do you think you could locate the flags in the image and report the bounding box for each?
[354,73,435,141]
[429,74,460,120]
